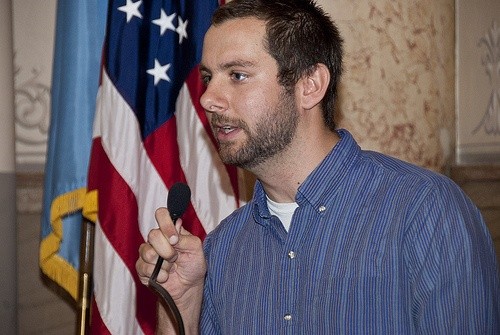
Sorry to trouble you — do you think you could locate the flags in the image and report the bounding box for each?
[83,0,241,335]
[37,0,109,303]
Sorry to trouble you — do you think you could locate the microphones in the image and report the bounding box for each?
[148,182,190,335]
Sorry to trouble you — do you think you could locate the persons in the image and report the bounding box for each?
[134,0,500,335]
